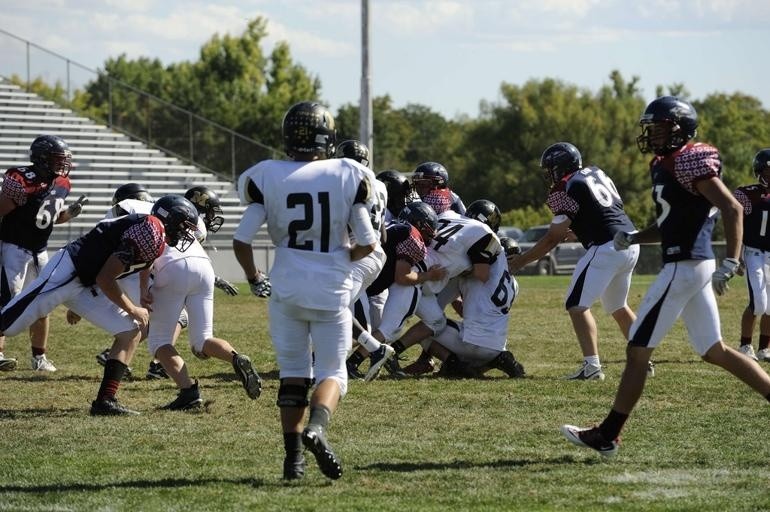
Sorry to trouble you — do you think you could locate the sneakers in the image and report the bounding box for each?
[0,351,19,372]
[562,424,620,459]
[346,342,525,384]
[90,350,262,416]
[565,359,606,382]
[756,348,770,360]
[301,425,343,480]
[282,458,305,481]
[737,344,758,361]
[31,353,57,372]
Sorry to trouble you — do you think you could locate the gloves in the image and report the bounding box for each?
[247,270,272,298]
[66,193,89,220]
[215,276,239,297]
[712,256,740,297]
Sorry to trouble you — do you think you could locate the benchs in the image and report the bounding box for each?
[0,75,279,249]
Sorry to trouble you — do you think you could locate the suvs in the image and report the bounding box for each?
[515,223,588,274]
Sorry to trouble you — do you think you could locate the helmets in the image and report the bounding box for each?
[636,95,699,157]
[499,236,521,260]
[538,141,584,188]
[110,183,225,253]
[282,100,337,162]
[335,139,502,247]
[751,148,769,186]
[27,134,73,178]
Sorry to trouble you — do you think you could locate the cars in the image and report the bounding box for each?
[495,226,523,240]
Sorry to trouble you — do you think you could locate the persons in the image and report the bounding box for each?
[560,96,770,457]
[0,135,90,373]
[104,183,262,411]
[230,98,525,482]
[0,194,200,416]
[506,141,656,383]
[728,150,770,367]
[95,186,239,381]
